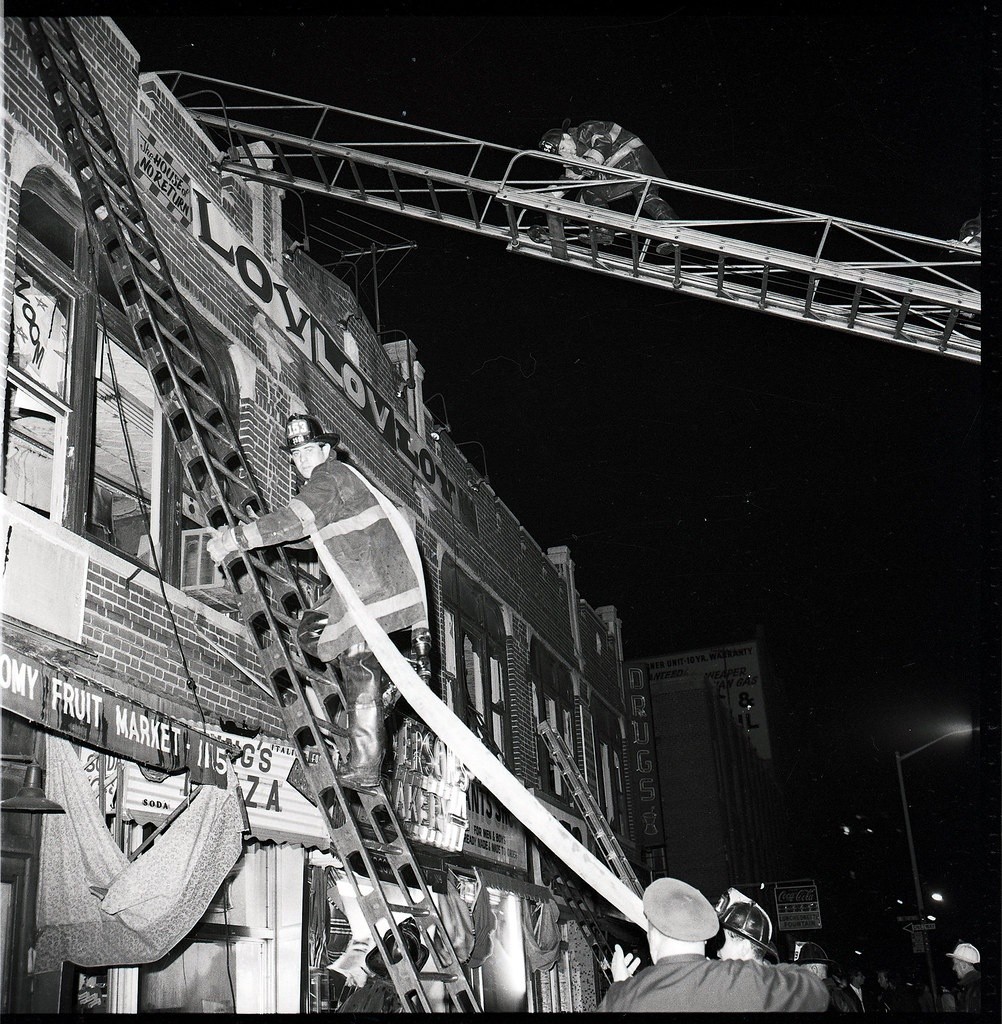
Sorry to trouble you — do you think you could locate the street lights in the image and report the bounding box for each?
[893,722,996,1012]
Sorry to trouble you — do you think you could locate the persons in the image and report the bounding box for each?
[206,412,428,788]
[539,121,688,254]
[598,876,995,1015]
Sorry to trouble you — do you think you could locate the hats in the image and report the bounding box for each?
[642,876,720,940]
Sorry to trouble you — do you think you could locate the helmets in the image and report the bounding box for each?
[782,940,836,970]
[715,888,780,966]
[946,943,980,964]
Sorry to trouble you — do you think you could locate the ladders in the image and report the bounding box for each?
[536,720,644,899]
[25,19,483,1013]
[445,672,616,988]
